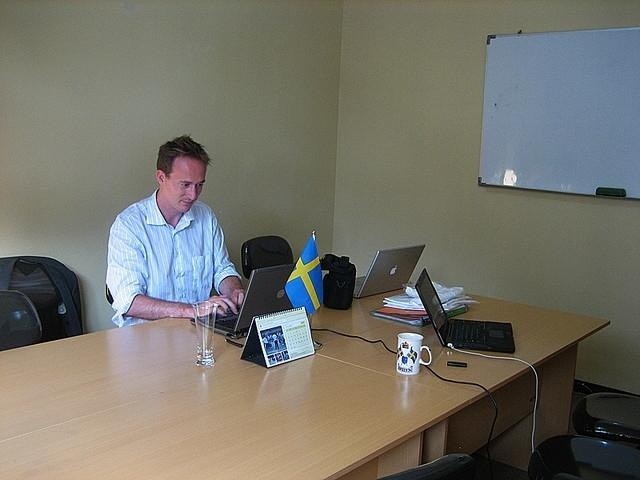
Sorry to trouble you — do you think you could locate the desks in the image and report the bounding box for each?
[308,283,612,465]
[1,318,469,480]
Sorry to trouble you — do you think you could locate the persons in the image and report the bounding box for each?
[105,136,245,328]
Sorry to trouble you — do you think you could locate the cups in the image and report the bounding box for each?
[395,332,433,376]
[192,301,218,368]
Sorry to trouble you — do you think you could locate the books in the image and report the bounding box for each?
[369,280,480,327]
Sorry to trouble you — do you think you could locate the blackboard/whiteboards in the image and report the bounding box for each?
[478,27,640,200]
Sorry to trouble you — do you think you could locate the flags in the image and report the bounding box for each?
[284,232,323,315]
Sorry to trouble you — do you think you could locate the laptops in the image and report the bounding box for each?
[354,243,427,301]
[189,264,294,341]
[414,265,517,355]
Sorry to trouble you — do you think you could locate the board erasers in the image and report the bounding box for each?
[596,187,626,197]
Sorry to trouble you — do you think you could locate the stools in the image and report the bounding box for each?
[572,389,640,443]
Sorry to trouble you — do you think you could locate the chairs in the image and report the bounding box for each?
[241,236,294,279]
[525,431,640,480]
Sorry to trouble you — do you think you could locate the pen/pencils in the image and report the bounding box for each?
[224,338,243,348]
